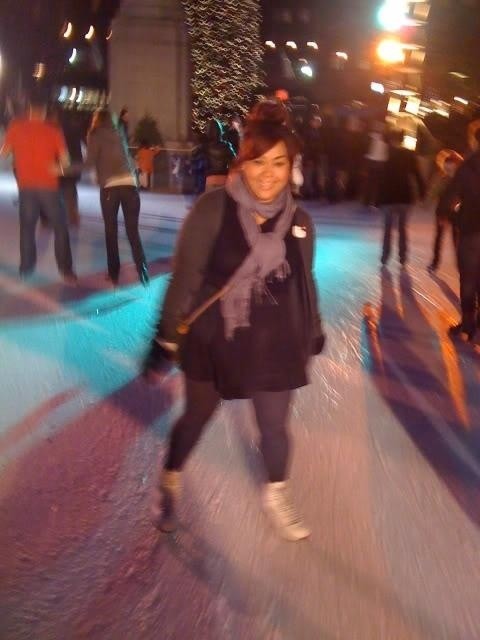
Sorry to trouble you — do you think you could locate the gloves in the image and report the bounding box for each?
[145,337,180,385]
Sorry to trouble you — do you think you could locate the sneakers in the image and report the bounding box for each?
[377,253,440,270]
[450,322,477,345]
[261,480,313,543]
[148,467,183,534]
[16,262,149,286]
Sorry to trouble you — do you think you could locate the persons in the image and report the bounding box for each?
[140,99,326,542]
